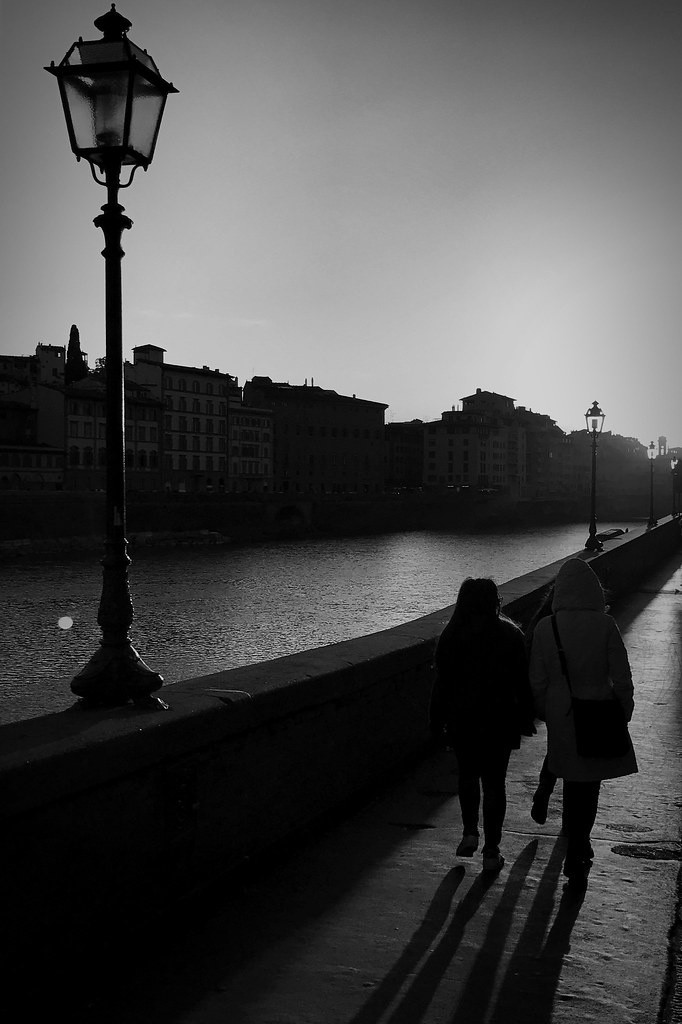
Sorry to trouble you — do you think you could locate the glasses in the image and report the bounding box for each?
[498,596,503,605]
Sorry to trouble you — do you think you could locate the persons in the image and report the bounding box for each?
[527,559,638,885]
[433,575,538,869]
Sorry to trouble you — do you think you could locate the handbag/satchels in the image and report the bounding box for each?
[570,697,630,759]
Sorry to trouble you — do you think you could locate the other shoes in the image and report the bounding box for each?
[456,833,477,857]
[564,849,594,878]
[483,854,504,874]
[531,785,550,825]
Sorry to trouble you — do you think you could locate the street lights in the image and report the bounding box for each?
[585,399,606,552]
[670,456,680,517]
[40,1,180,717]
[645,441,658,529]
